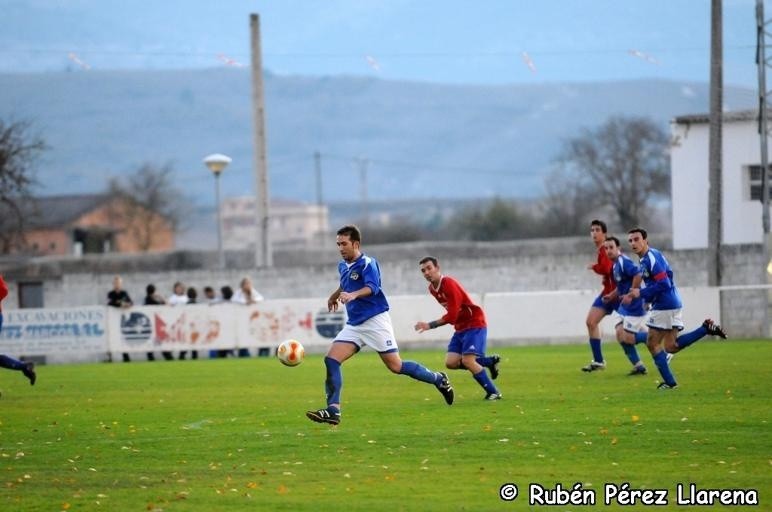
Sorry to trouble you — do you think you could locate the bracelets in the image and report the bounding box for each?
[429,321,439,328]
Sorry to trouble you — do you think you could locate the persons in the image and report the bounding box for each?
[413,256,502,400]
[203,276,271,358]
[142,283,175,360]
[179,287,199,360]
[598,237,651,375]
[0,271,37,386]
[106,274,134,364]
[304,223,454,424]
[627,226,729,391]
[582,219,674,374]
[167,281,189,306]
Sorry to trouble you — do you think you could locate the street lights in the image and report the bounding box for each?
[204,152,234,268]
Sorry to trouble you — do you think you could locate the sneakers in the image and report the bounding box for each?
[657,382,677,391]
[627,367,647,375]
[490,356,499,379]
[703,319,728,339]
[307,409,340,425]
[437,372,454,404]
[483,392,502,400]
[23,362,36,385]
[582,360,606,372]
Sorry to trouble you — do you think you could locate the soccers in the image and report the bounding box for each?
[277,340,304,366]
